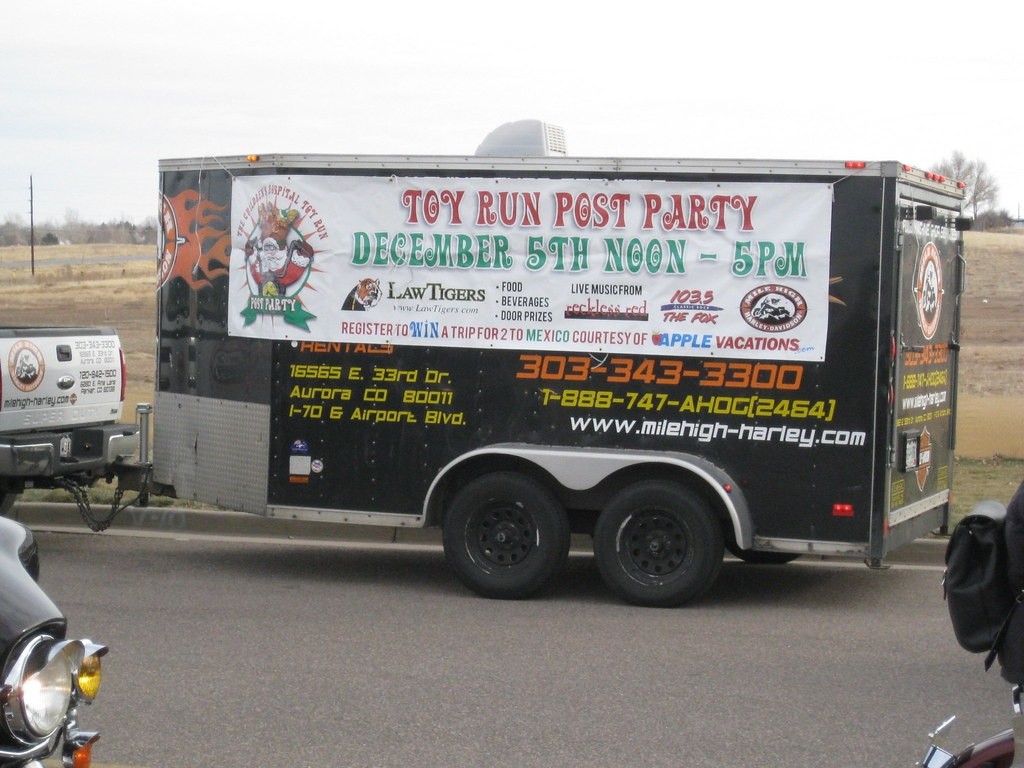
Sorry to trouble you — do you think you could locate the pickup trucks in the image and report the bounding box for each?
[0,322,143,520]
